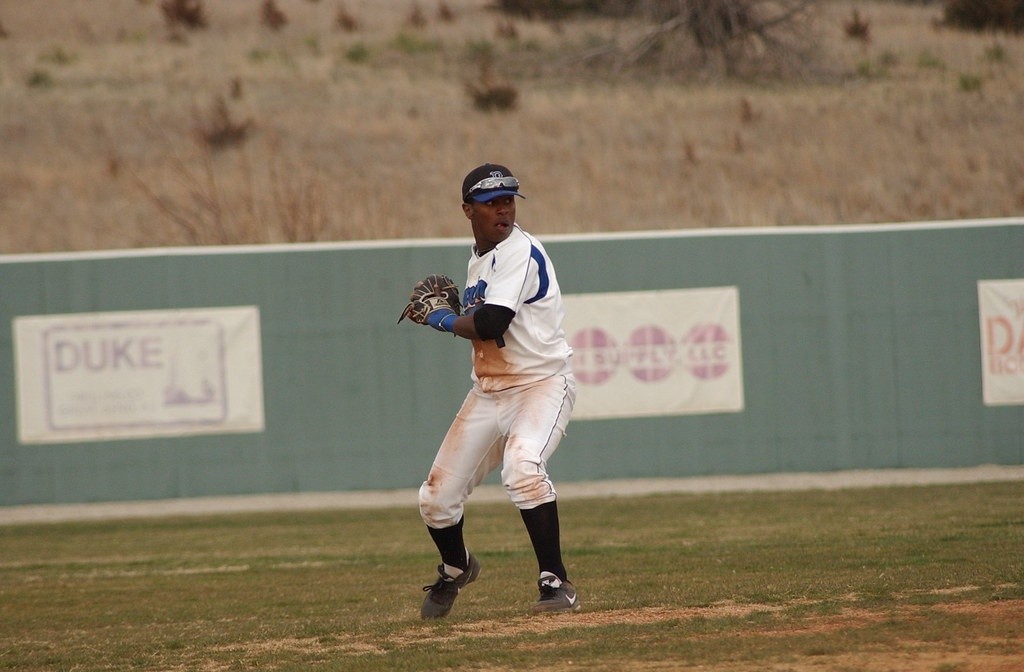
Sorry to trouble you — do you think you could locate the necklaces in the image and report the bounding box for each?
[476,247,494,252]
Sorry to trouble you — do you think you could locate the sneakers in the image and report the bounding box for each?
[534,571,581,615]
[421,551,481,620]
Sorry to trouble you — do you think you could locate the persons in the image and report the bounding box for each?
[396,165,579,621]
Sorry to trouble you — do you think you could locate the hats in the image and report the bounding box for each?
[462,163,526,202]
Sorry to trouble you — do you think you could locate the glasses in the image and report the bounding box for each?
[464,177,519,200]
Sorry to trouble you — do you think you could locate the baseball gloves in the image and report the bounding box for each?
[402,274,462,324]
